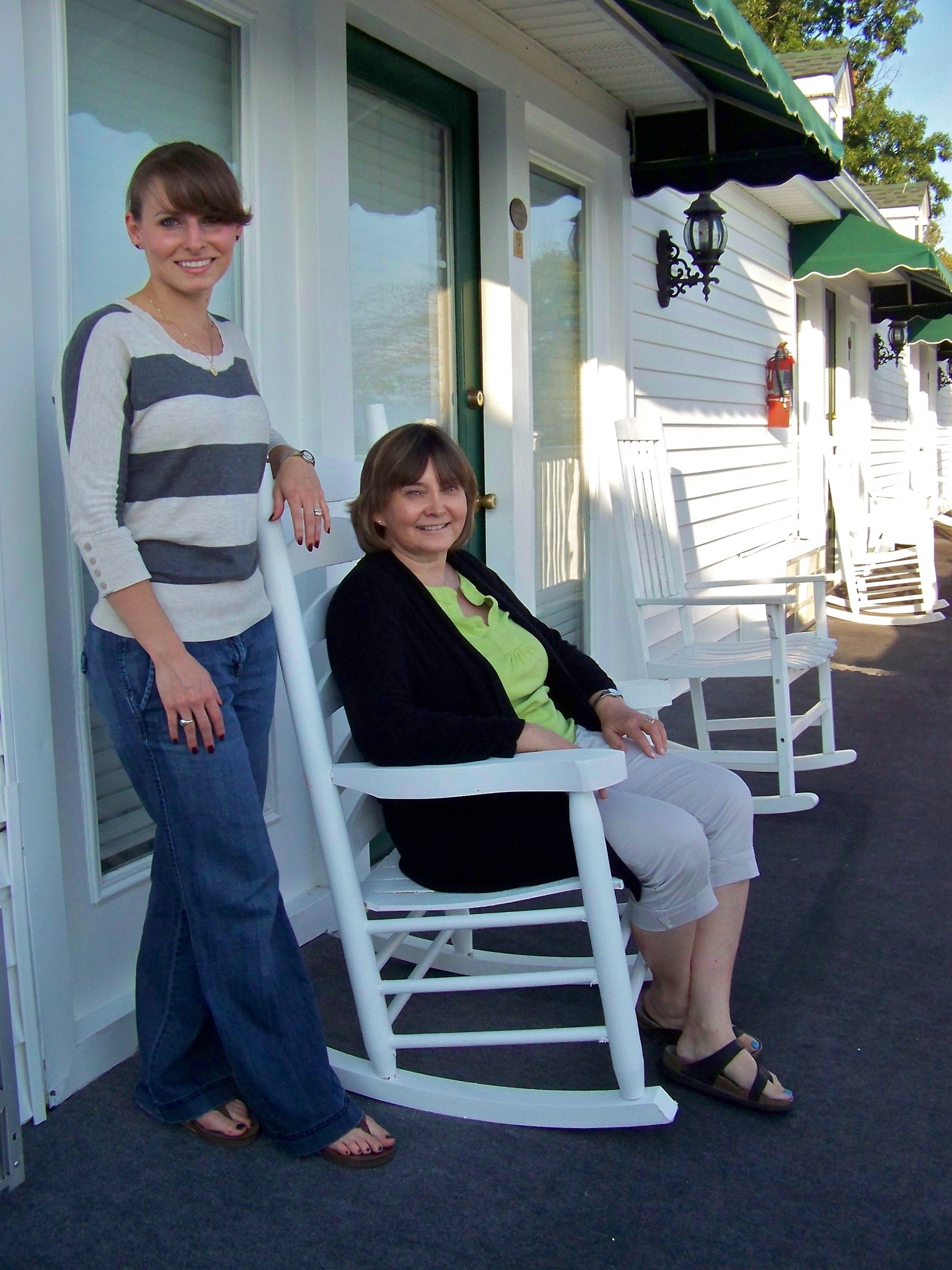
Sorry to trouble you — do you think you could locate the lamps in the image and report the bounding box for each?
[874,319,908,368]
[656,190,728,308]
[937,358,952,389]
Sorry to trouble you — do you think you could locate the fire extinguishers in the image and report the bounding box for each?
[766,341,793,427]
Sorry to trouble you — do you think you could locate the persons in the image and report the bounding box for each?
[325,423,798,1114]
[52,141,403,1170]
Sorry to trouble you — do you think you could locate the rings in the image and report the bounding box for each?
[313,509,323,515]
[646,716,659,723]
[179,719,193,727]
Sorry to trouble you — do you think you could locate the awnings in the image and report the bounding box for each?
[907,314,952,361]
[613,0,846,199]
[788,209,952,324]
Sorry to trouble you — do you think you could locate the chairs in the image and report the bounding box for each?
[823,438,949,626]
[259,403,678,1130]
[606,421,856,813]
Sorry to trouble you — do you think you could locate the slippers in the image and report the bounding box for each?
[318,1113,396,1169]
[663,1039,794,1109]
[635,991,762,1057]
[178,1099,260,1148]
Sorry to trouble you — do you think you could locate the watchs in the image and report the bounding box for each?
[278,449,315,467]
[591,688,624,709]
